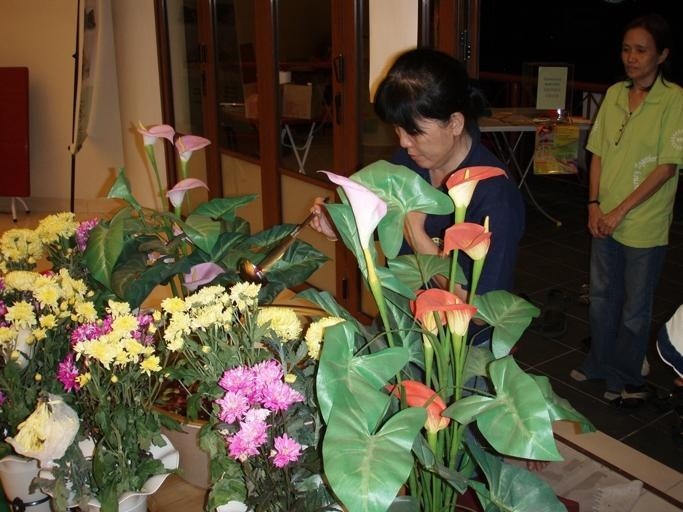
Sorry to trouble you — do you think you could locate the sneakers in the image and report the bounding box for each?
[538,288,570,339]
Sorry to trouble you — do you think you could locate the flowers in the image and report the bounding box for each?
[1,120,507,467]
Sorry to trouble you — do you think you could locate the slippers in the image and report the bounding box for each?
[609,383,654,414]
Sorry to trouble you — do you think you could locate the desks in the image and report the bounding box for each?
[478,108,593,227]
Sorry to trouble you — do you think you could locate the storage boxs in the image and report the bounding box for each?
[243,82,329,121]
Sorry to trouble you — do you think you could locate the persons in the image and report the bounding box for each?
[306,48,526,490]
[568,15,682,402]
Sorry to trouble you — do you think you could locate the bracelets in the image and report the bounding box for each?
[585,199,601,206]
[326,236,341,242]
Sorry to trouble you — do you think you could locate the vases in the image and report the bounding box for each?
[1,453,52,512]
[215,473,347,511]
[147,403,215,490]
[39,428,181,512]
[405,479,580,512]
